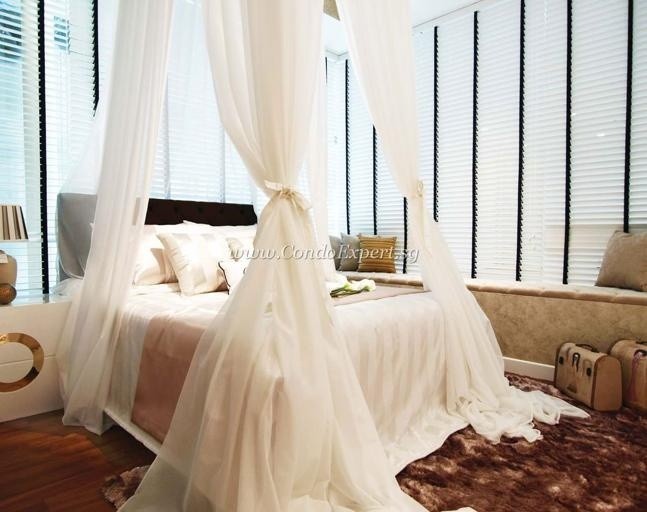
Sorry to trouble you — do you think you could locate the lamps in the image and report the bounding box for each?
[0,203,28,284]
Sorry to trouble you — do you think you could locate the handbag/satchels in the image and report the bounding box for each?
[554,340,623,412]
[607,338,647,413]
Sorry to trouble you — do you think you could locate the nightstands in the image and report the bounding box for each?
[0,294,70,422]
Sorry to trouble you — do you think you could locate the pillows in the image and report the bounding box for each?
[327,231,397,274]
[594,229,647,291]
[132,220,258,296]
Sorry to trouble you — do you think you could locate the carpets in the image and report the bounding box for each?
[98,371,647,510]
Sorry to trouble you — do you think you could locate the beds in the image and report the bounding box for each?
[57,191,480,511]
